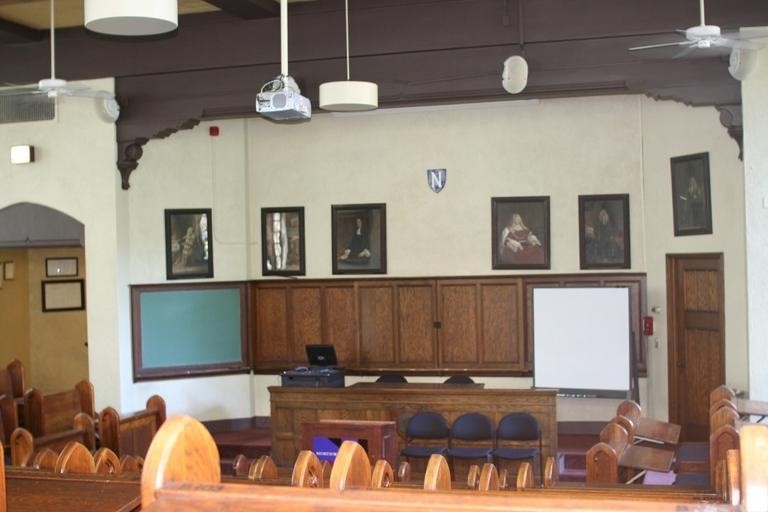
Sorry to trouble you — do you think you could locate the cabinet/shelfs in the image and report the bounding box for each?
[358,278,439,374]
[438,277,533,377]
[249,279,359,375]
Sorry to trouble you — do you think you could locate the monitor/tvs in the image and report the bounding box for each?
[305,345,337,366]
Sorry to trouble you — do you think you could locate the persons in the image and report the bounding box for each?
[678,175,705,223]
[584,210,609,263]
[593,207,618,258]
[338,212,372,265]
[500,209,543,262]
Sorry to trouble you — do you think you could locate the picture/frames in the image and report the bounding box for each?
[39,279,85,313]
[331,202,388,274]
[45,257,80,278]
[669,152,713,237]
[576,193,633,270]
[490,196,552,271]
[260,207,305,277]
[164,208,215,278]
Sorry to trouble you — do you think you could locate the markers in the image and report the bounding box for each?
[556,394,598,398]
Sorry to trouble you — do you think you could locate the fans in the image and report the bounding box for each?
[626,0,767,63]
[0,0,117,102]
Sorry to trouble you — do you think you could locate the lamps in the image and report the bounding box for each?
[83,0,178,37]
[318,1,379,113]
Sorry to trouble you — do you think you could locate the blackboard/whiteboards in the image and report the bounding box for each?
[532,286,634,400]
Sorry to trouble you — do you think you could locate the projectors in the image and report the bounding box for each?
[255,91,312,121]
